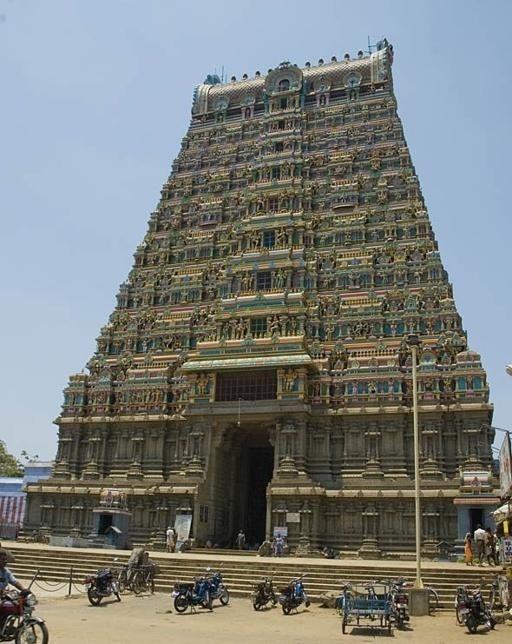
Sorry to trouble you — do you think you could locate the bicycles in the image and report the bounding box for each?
[424,586,439,612]
[81,562,156,606]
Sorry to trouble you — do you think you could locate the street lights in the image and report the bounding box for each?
[405,334,424,590]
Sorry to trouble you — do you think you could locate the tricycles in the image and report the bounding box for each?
[342,582,391,634]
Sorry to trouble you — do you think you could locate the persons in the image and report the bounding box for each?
[464,524,499,567]
[0,550,31,595]
[236,530,245,550]
[275,534,283,556]
[324,546,337,559]
[166,526,178,552]
[143,552,156,567]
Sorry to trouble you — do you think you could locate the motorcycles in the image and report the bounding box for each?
[249,567,309,614]
[455,585,496,634]
[391,579,412,627]
[174,562,229,614]
[0,589,48,644]
